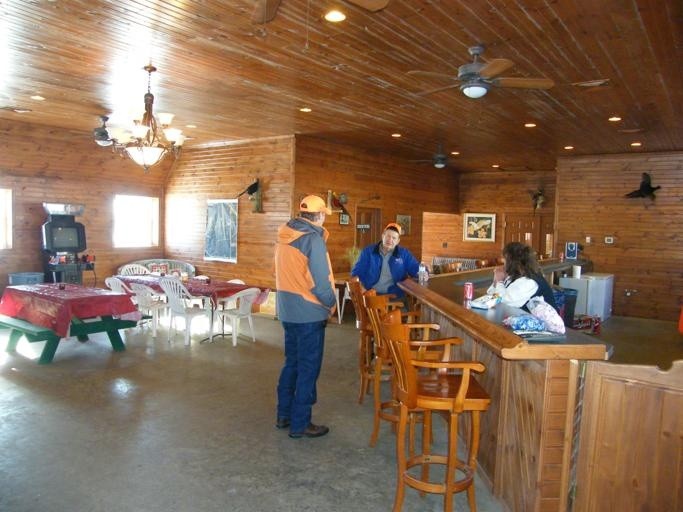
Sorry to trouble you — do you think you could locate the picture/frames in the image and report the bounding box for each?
[461,213,497,243]
[396,214,411,236]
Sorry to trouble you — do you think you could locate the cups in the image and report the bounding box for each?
[572,265,581,279]
[206,276,211,284]
[160,266,166,276]
[59,283,65,290]
[181,272,189,286]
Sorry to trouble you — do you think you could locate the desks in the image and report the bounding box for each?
[325,272,355,323]
[1,281,137,364]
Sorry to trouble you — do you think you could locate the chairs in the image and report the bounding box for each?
[106,258,271,347]
[345,274,493,512]
[432,261,462,275]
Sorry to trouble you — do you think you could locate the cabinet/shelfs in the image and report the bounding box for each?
[48,260,83,285]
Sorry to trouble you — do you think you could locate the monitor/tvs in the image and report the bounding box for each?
[41,221,87,253]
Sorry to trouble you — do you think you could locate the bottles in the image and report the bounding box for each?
[419,260,426,282]
[558,242,565,262]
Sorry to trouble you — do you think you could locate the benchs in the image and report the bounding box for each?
[2,315,53,337]
[70,319,137,343]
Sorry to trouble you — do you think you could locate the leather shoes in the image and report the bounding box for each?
[276,418,329,438]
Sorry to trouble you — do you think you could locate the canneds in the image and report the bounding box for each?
[465,281,476,298]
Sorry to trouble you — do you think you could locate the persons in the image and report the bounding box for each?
[275,195,336,437]
[487,241,561,316]
[351,222,429,324]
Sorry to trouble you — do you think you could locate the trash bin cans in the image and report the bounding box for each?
[9,271,44,284]
[565,289,577,327]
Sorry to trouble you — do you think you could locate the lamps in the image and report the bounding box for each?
[94,63,185,172]
[433,161,446,169]
[460,73,488,99]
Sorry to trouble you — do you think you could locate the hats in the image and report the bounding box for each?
[383,222,401,235]
[300,195,332,215]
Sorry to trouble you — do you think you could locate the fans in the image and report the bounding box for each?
[246,0,391,25]
[405,46,556,99]
[408,143,472,170]
